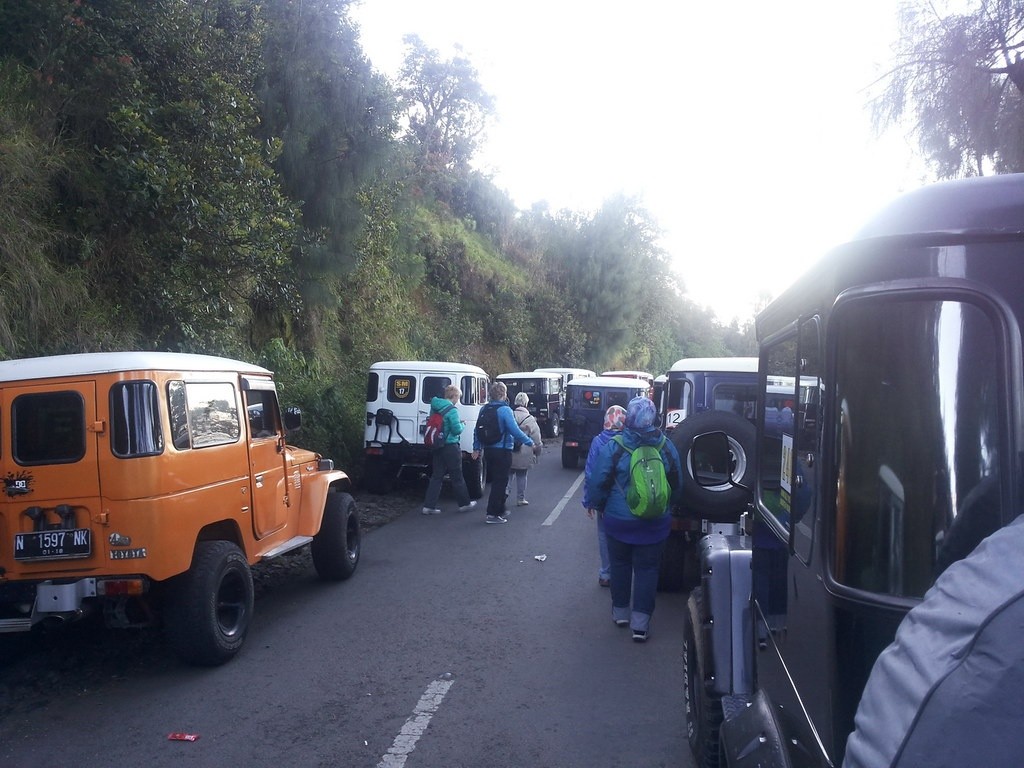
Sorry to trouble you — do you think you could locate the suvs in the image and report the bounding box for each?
[0,350,361,666]
[599,371,653,386]
[653,357,763,588]
[766,376,820,438]
[562,378,651,469]
[355,361,490,496]
[496,372,565,436]
[534,368,595,388]
[684,167,1024,767]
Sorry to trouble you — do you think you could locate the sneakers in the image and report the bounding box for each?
[500,510,511,518]
[485,515,508,524]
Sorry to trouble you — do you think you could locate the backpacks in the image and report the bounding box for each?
[610,435,672,521]
[423,405,459,449]
[512,410,531,451]
[476,403,508,444]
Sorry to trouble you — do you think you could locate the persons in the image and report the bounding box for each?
[504,392,543,506]
[582,396,682,640]
[839,512,1024,767]
[471,382,537,524]
[422,385,477,516]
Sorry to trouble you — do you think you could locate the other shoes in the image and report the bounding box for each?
[517,500,529,505]
[631,630,649,641]
[422,507,442,514]
[460,501,478,513]
